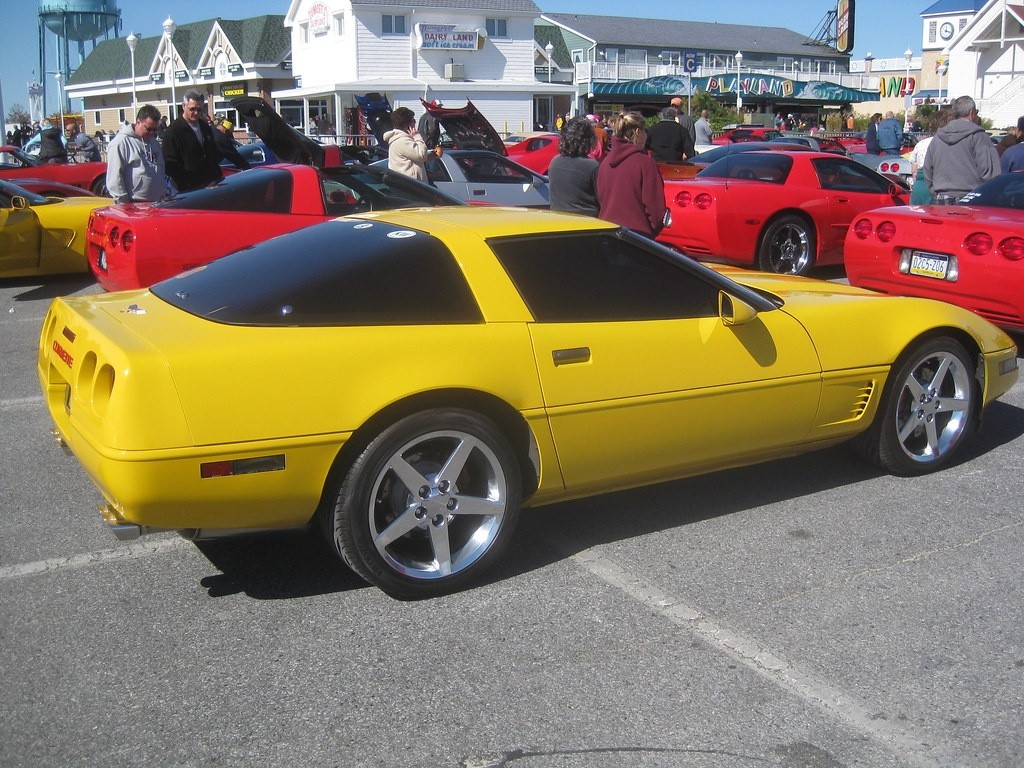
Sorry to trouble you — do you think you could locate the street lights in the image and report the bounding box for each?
[161,15,178,121]
[903,49,913,131]
[734,50,743,117]
[125,30,138,123]
[545,42,554,133]
[54,73,65,136]
[936,64,945,111]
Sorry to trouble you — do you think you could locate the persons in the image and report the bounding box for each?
[921,96,1000,205]
[382,107,439,185]
[7,116,251,171]
[309,114,335,135]
[417,99,444,149]
[106,104,168,205]
[773,112,1024,205]
[160,88,225,199]
[533,95,713,246]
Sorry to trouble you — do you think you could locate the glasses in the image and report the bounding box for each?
[878,117,882,121]
[408,118,417,125]
[139,119,158,132]
[184,103,203,113]
[634,127,649,135]
[968,108,980,115]
[65,127,72,132]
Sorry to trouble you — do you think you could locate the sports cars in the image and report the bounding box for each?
[36,205,1020,599]
[81,161,501,291]
[0,177,118,280]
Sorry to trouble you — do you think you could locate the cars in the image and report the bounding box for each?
[651,151,911,279]
[0,144,111,197]
[840,168,1024,334]
[209,94,707,256]
[647,125,921,183]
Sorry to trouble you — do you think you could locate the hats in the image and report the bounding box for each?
[220,120,235,139]
[671,98,682,107]
[788,113,793,118]
